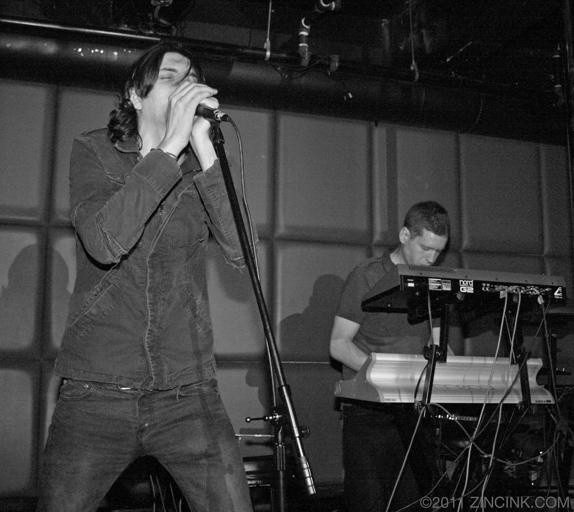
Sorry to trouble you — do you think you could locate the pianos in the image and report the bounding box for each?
[334,352,556,409]
[360,263,568,315]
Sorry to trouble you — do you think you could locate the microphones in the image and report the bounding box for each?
[194,103,231,122]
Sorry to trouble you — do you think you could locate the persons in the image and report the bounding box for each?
[36,40,257,512]
[327,200,454,511]
